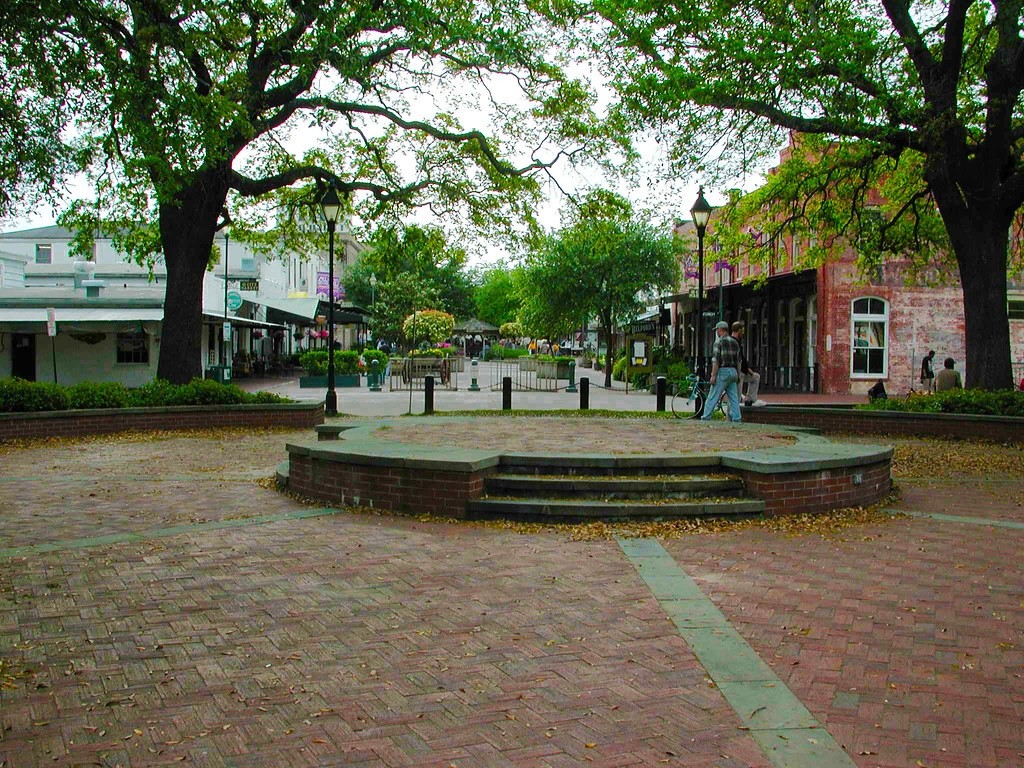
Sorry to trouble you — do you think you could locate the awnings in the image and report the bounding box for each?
[0,306,163,336]
[202,312,287,331]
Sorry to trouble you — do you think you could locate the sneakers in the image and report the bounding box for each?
[752,398,767,406]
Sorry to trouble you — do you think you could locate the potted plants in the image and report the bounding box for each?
[298,351,389,388]
[293,332,305,342]
[519,342,605,379]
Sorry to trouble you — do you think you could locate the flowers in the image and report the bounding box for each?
[307,328,318,338]
[274,331,285,340]
[318,330,328,339]
[252,330,263,340]
[326,331,330,338]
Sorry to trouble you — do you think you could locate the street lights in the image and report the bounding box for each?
[220,220,233,369]
[688,183,712,420]
[370,273,377,349]
[319,179,340,418]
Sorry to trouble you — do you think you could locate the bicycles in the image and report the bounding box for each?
[671,372,727,419]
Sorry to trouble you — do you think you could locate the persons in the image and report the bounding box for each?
[920,350,935,392]
[360,335,391,379]
[700,321,760,423]
[936,358,962,392]
[438,334,560,357]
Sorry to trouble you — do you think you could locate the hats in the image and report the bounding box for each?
[945,358,957,364]
[712,321,728,330]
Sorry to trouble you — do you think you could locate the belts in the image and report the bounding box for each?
[720,365,737,368]
